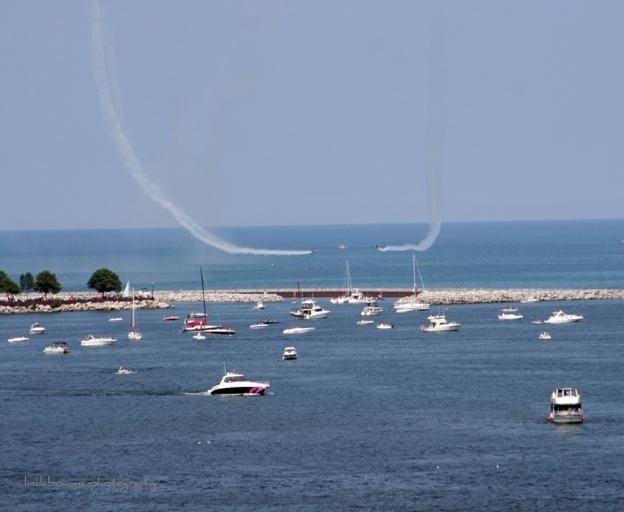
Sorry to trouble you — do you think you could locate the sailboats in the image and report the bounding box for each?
[182,265,235,339]
[393,253,431,313]
[128,286,143,342]
[330,260,384,305]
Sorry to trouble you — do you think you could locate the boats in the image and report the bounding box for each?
[108,317,122,323]
[81,334,118,348]
[8,336,30,344]
[193,333,206,341]
[187,312,207,318]
[283,326,316,335]
[419,314,462,332]
[289,298,331,319]
[115,365,136,375]
[207,370,270,396]
[530,309,584,340]
[356,306,394,329]
[248,300,277,330]
[283,346,298,360]
[546,387,587,423]
[162,314,179,321]
[29,321,45,335]
[43,343,70,355]
[497,306,524,321]
